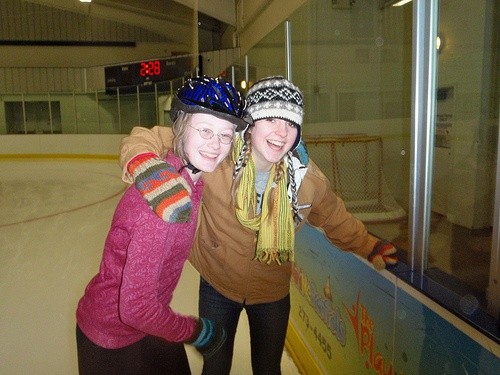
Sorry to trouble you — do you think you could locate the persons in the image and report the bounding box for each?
[119,75,399,375]
[75,76,248,375]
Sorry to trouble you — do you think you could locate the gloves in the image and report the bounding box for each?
[187,317,227,358]
[291,137,309,167]
[367,239,397,270]
[127,152,192,224]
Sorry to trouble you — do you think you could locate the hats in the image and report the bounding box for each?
[231,74,303,224]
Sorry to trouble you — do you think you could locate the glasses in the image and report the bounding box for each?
[185,122,235,145]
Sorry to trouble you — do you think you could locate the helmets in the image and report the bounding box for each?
[170,75,254,132]
